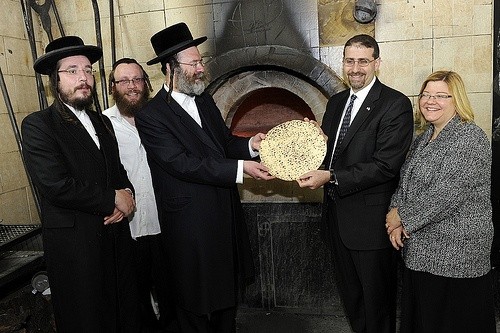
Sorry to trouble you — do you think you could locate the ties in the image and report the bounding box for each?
[327,94,358,199]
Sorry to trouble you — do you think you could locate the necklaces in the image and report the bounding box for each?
[429,138,434,144]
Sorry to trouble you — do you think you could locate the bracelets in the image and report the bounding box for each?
[329,169,335,184]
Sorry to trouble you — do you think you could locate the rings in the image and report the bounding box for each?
[391,236,395,239]
[385,223,388,228]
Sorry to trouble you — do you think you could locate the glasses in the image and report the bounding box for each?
[57,66,94,77]
[177,59,206,69]
[418,91,453,102]
[114,78,145,86]
[342,57,379,67]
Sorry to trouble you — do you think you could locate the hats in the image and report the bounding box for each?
[33,36,103,74]
[146,22,207,65]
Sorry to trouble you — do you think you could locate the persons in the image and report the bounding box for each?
[385,70,495,333]
[132,22,278,333]
[296,33,415,332]
[20,35,153,333]
[101,58,175,333]
